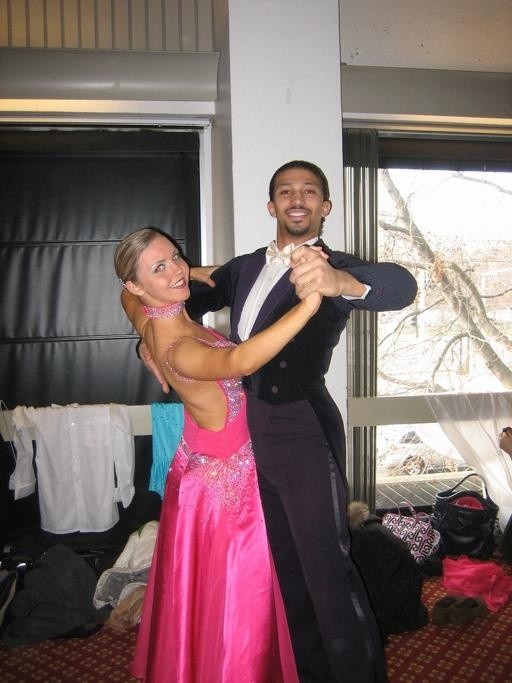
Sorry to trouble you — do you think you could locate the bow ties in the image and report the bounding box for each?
[265,241,295,267]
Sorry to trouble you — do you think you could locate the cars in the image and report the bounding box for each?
[385,429,465,476]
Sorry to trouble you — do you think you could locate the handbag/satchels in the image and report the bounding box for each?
[351,512,430,638]
[0,489,163,649]
[380,513,441,564]
[433,473,500,562]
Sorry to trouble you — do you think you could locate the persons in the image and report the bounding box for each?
[497,428,512,460]
[134,159,422,683]
[112,226,328,682]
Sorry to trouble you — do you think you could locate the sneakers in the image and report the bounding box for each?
[432,595,460,625]
[450,597,486,627]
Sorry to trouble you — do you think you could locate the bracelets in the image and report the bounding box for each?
[135,335,146,362]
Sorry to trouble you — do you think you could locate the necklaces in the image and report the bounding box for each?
[138,299,186,319]
